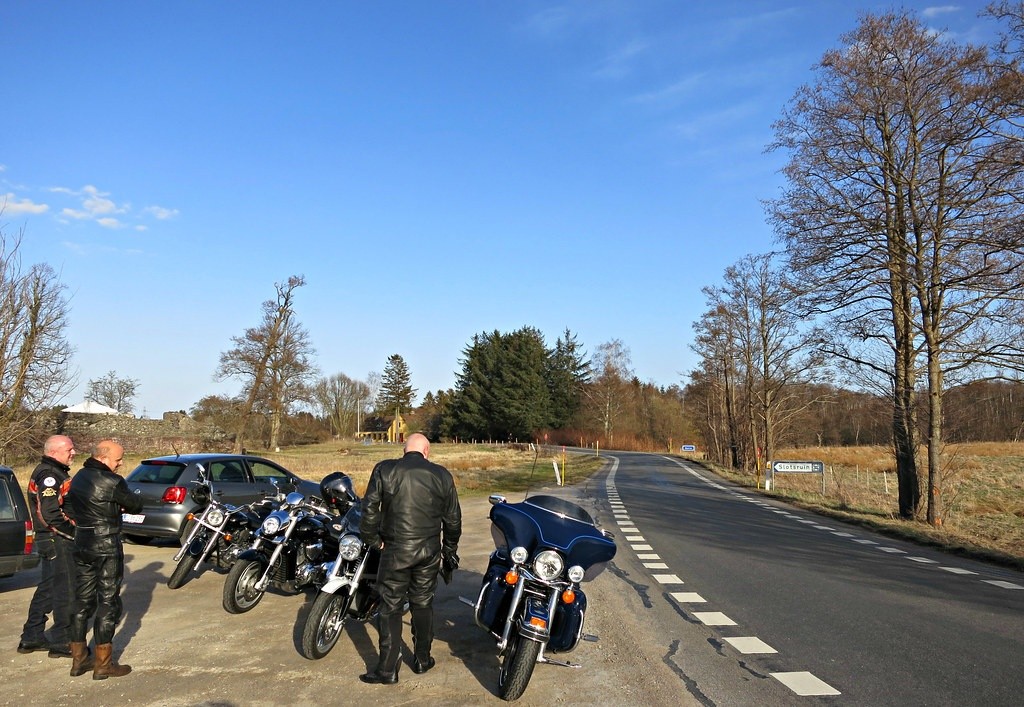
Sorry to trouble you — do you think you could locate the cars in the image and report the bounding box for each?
[119,453,323,548]
[0,466,41,579]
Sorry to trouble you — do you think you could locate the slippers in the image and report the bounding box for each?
[71,641,95,676]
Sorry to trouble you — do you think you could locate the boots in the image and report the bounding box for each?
[94,643,132,680]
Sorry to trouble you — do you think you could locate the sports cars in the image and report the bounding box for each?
[472,494,619,701]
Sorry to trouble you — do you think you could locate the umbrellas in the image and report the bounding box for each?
[62,397,120,417]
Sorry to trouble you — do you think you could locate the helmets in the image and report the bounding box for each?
[320,470,351,509]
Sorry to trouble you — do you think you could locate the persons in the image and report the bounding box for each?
[359,431,464,686]
[67,441,133,682]
[15,435,92,660]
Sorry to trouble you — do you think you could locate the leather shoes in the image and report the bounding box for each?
[412,656,435,673]
[359,669,397,684]
[49,647,91,658]
[17,638,51,654]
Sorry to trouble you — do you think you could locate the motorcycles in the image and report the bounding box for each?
[221,477,341,615]
[165,462,289,590]
[300,485,410,660]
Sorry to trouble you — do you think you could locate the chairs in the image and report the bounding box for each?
[220,466,244,483]
